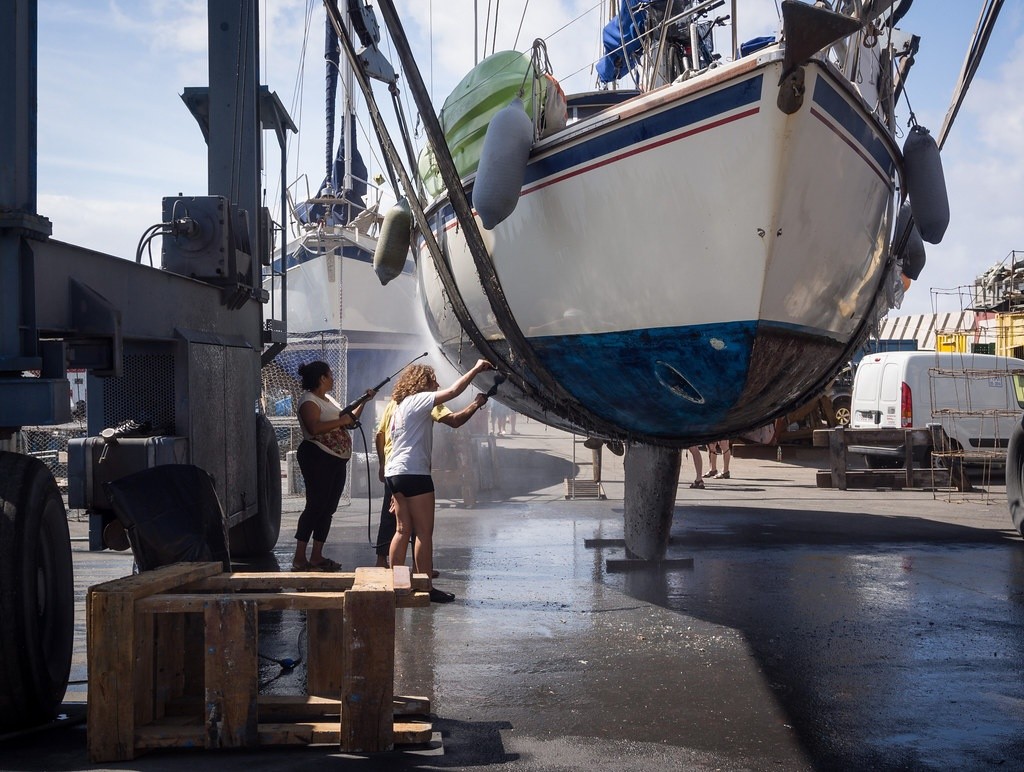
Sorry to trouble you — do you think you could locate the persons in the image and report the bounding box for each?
[688,439,731,489]
[374,359,495,602]
[292,360,378,571]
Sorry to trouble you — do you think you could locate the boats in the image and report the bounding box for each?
[405,1,955,452]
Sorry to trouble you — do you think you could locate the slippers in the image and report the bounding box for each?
[291,561,323,572]
[308,558,341,570]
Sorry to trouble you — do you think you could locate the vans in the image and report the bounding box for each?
[856,353,1024,480]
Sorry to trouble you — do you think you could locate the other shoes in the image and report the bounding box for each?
[410,588,455,603]
[412,567,440,578]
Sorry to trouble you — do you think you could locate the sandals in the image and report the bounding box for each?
[702,470,718,478]
[712,471,730,479]
[690,479,705,489]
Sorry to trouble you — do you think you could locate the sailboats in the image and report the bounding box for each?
[262,0,494,460]
